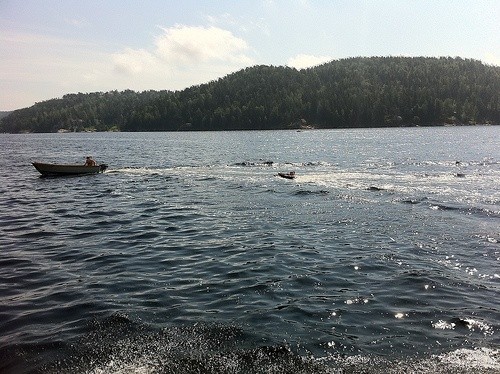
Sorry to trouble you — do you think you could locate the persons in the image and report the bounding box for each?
[83,156,96,166]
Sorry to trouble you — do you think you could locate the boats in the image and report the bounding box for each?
[278,170,296,178]
[30,160,109,176]
